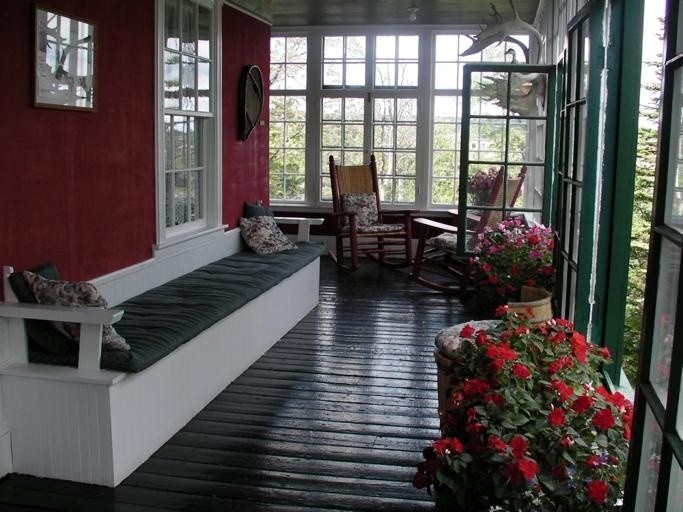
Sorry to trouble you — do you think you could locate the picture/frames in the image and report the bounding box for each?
[32,3,99,113]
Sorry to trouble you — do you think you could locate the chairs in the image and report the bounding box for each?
[433,285,553,435]
[409,165,527,295]
[328,155,420,272]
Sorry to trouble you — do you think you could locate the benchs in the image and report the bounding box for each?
[0,216,326,488]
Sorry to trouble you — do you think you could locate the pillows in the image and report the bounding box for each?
[9,261,76,356]
[244,202,274,218]
[239,216,298,256]
[340,192,380,226]
[23,270,131,351]
[433,232,472,250]
